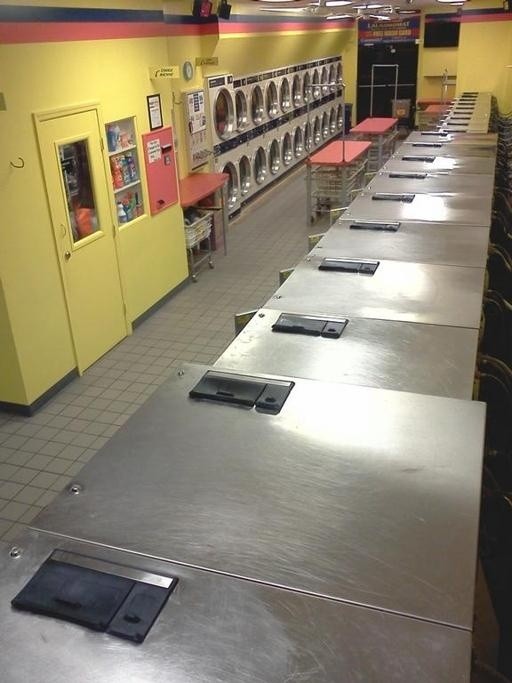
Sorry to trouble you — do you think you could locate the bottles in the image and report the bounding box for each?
[107,125,128,151]
[117,192,142,224]
[110,151,139,189]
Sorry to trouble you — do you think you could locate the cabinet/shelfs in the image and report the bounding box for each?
[104,114,148,232]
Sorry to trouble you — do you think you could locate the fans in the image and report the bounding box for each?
[258,0,420,22]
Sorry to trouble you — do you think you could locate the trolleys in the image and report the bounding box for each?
[302,158,366,227]
[183,209,216,283]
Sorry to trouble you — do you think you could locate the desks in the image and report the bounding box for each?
[0,527,512,682]
[30,362,511,630]
[211,309,510,400]
[304,91,512,269]
[179,172,229,276]
[263,256,512,329]
